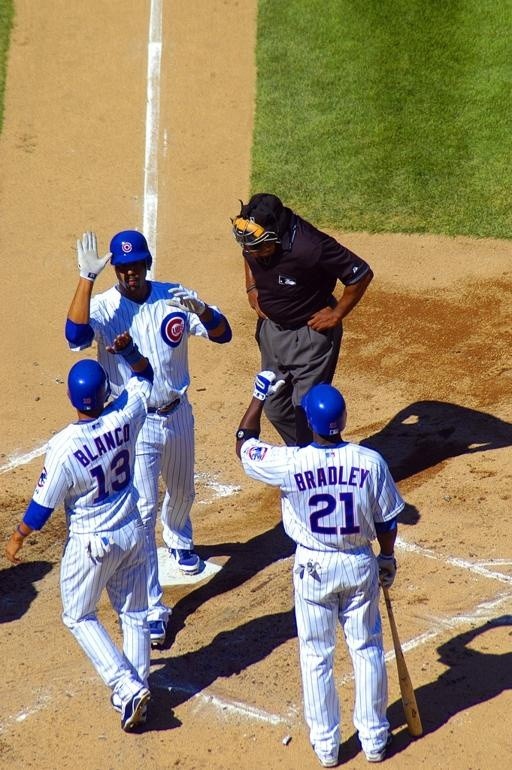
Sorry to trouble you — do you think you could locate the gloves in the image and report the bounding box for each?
[253,370,286,401]
[295,561,316,575]
[166,288,206,317]
[84,533,115,558]
[75,229,113,282]
[377,552,396,589]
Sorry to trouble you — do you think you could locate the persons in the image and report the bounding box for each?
[65,231,232,577]
[235,370,406,768]
[4,331,154,731]
[232,193,373,446]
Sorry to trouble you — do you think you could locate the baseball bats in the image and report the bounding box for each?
[380,574,423,736]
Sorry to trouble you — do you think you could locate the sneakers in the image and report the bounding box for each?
[313,744,339,767]
[365,730,392,763]
[164,541,200,575]
[121,686,153,731]
[111,693,148,723]
[148,620,168,647]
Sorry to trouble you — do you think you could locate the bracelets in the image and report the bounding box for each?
[247,284,256,293]
[16,525,28,537]
[121,346,143,366]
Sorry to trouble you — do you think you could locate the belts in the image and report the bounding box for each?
[148,399,181,416]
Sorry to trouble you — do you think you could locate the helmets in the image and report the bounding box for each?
[303,384,347,436]
[68,359,112,413]
[110,230,153,271]
[230,194,286,265]
